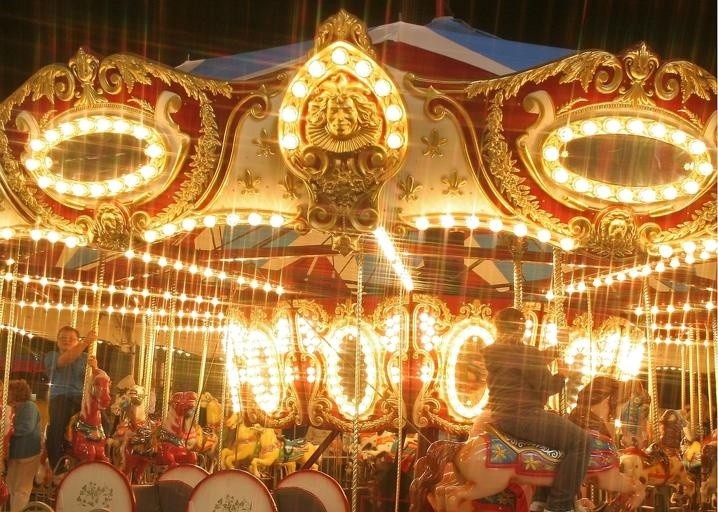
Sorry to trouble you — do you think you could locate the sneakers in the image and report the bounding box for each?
[53,463,69,477]
[544,501,576,512]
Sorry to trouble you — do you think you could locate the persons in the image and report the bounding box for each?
[480,307,593,512]
[45,325,98,481]
[7,379,41,512]
